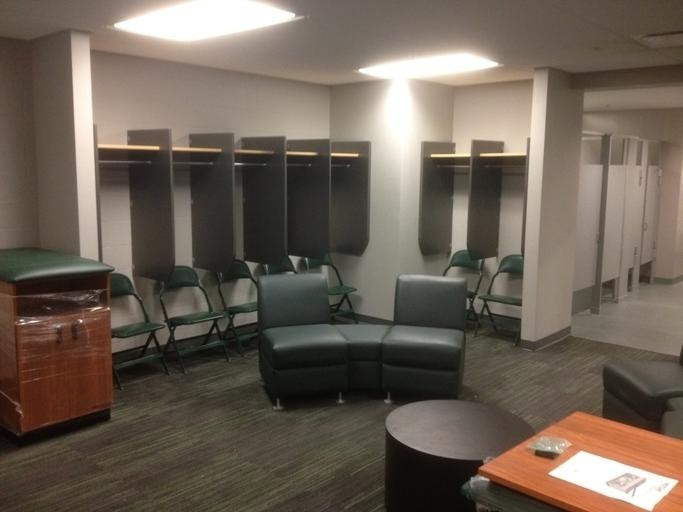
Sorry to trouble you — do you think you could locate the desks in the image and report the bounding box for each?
[382,397,535,510]
[463,409,682,510]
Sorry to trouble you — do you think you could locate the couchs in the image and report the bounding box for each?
[255,273,468,406]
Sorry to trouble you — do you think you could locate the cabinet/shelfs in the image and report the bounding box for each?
[0,279,112,444]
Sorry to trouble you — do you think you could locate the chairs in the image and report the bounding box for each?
[472,254,524,346]
[214,259,260,359]
[441,249,484,329]
[301,250,359,325]
[262,255,297,274]
[603,344,682,439]
[156,264,231,375]
[107,272,169,392]
